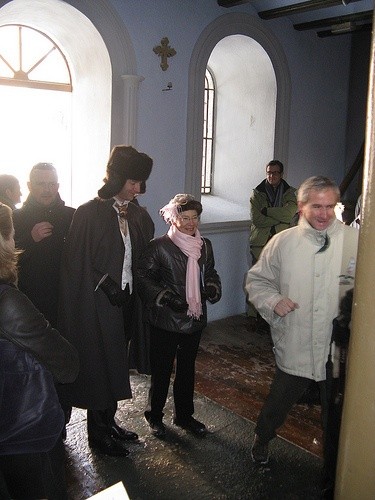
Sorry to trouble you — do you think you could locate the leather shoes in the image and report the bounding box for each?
[111,423,138,440]
[89,438,130,460]
[174,417,205,433]
[149,420,167,436]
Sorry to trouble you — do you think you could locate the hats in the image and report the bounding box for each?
[161,194,203,221]
[98,145,152,199]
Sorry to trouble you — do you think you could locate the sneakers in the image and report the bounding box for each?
[251,432,271,464]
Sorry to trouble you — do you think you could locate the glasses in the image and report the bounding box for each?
[182,216,199,223]
[267,171,281,176]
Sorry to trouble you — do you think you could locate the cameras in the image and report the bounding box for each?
[332,289,353,343]
